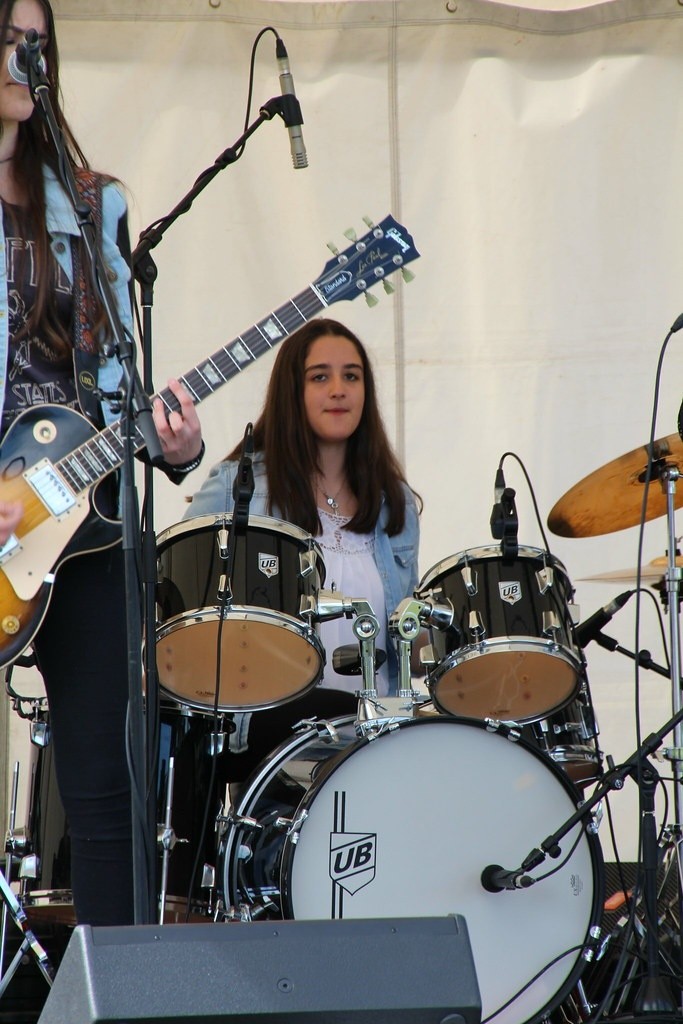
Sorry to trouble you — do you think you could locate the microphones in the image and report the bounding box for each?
[8,27,47,83]
[275,38,309,168]
[232,424,255,501]
[481,864,533,893]
[572,590,633,649]
[489,469,506,539]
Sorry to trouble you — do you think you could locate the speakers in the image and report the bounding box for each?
[554,861,683,1024]
[38,914,482,1024]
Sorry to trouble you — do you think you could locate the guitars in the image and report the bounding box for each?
[0,209,425,676]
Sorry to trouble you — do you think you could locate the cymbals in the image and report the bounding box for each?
[572,554,683,583]
[547,431,683,541]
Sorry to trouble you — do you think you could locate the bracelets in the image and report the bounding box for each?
[161,438,205,473]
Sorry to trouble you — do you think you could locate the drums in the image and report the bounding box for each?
[191,695,609,1024]
[144,509,330,716]
[516,677,607,792]
[413,542,588,728]
[15,704,239,928]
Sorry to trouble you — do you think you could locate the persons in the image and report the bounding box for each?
[0,0,202,926]
[182,319,420,783]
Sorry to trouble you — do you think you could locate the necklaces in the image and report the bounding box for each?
[310,471,347,516]
